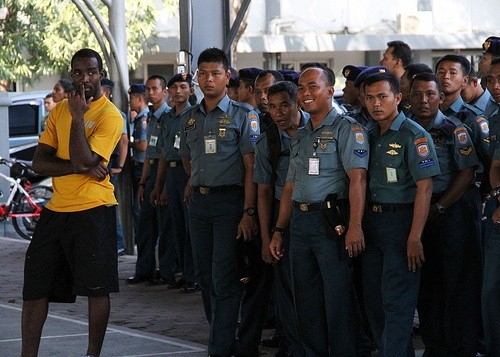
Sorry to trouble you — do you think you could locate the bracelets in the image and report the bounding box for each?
[119,167,123,169]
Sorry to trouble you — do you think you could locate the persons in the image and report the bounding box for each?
[38,36,500,357]
[268,68,372,357]
[180,47,262,357]
[21,48,125,357]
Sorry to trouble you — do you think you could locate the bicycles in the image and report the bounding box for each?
[0,156,53,240]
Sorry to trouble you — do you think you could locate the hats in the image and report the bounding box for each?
[277,70,299,86]
[342,65,369,81]
[355,66,388,87]
[128,85,145,93]
[166,73,192,88]
[226,76,240,88]
[239,68,262,81]
[482,36,500,53]
[100,78,115,87]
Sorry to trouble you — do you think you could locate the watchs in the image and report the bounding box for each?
[436,202,445,214]
[271,226,285,234]
[243,207,256,216]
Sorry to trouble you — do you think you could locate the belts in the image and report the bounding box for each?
[135,161,144,166]
[292,199,325,212]
[148,158,160,165]
[166,160,183,168]
[371,200,414,213]
[192,184,240,194]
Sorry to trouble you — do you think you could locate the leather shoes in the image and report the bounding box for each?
[125,275,154,284]
[276,351,291,357]
[261,339,278,348]
[167,279,187,289]
[144,278,176,286]
[181,282,202,293]
